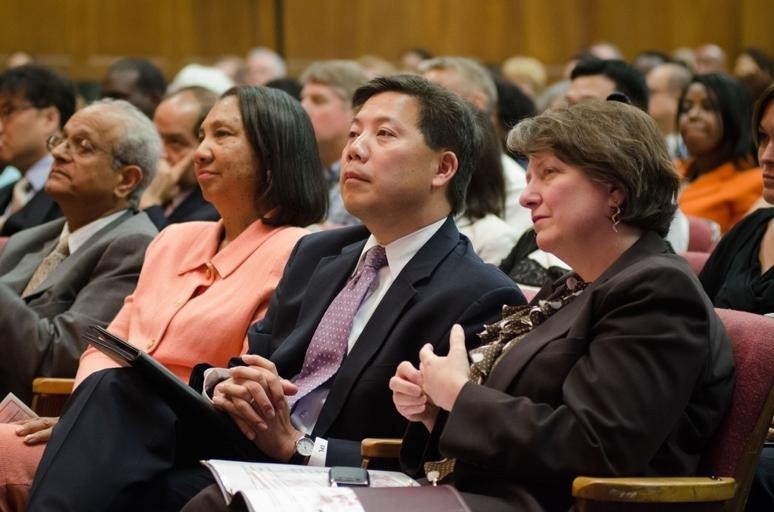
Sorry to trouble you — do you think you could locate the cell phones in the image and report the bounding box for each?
[328,466,370,488]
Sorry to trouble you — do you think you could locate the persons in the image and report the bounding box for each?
[0,40,774,511]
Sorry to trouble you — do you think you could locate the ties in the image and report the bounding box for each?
[0,179,30,233]
[283,245,389,431]
[20,236,70,301]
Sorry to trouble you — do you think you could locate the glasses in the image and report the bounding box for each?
[44,135,128,167]
[2,102,37,120]
